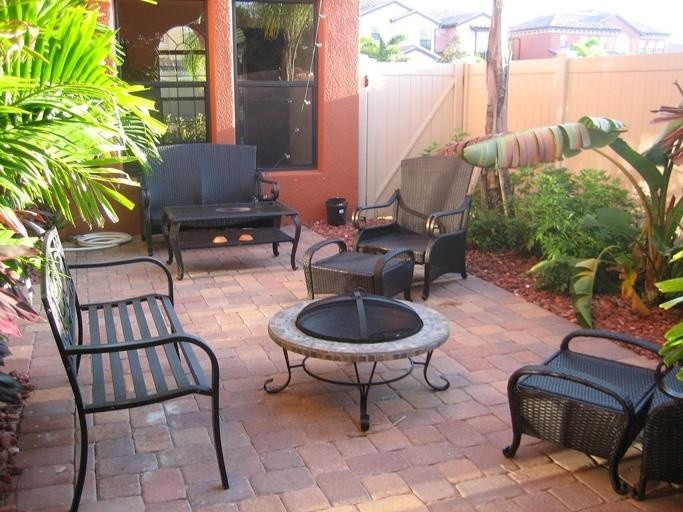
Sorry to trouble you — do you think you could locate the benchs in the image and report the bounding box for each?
[135,143,281,258]
[43,226,231,511]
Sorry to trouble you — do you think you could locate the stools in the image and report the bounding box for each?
[501,328,673,501]
[306,236,414,308]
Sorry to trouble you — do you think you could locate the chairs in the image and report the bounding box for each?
[297,153,480,301]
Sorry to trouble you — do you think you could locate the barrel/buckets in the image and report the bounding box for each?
[325,197,348,226]
